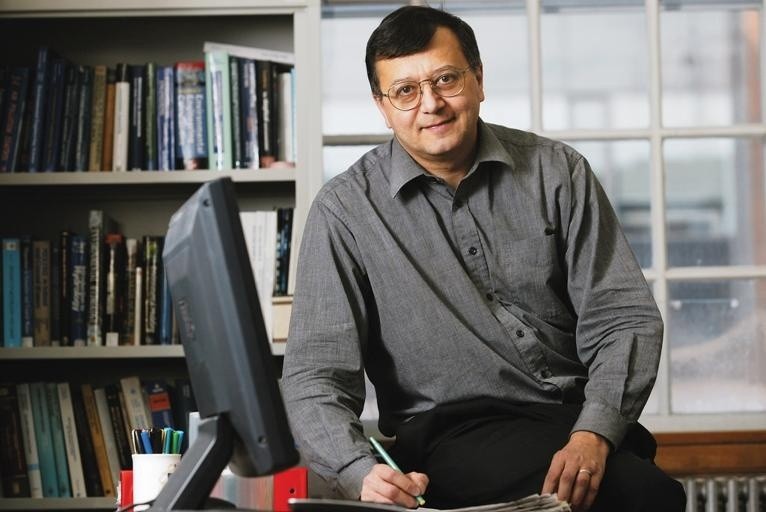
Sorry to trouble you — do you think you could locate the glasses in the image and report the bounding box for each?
[377,65,472,112]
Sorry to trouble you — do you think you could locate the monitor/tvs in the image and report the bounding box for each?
[138,174,301,512]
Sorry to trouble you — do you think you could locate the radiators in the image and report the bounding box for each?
[680,473,765,511]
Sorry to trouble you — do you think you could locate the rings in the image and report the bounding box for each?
[579,470,592,477]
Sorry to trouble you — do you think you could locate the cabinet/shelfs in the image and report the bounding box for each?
[0,0,323,510]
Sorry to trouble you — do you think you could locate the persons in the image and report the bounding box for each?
[278,6,686,512]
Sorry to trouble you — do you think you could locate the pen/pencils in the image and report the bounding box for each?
[131,427,184,454]
[369,436,427,506]
